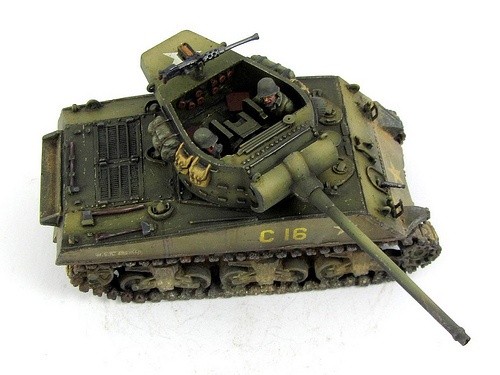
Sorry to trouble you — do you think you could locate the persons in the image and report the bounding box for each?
[251,77,295,125]
[191,126,223,159]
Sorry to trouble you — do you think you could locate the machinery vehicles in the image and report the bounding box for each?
[40,28,471,350]
